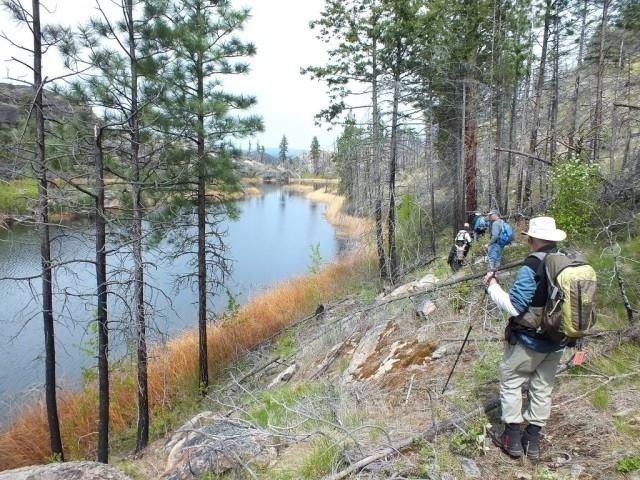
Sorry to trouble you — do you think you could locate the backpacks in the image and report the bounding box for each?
[529,248,599,348]
[495,219,514,247]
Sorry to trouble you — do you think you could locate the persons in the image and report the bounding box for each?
[455,223,472,243]
[470,210,489,242]
[482,216,567,458]
[483,209,505,271]
[447,235,471,274]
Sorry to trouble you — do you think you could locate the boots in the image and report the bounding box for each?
[520,430,540,460]
[494,429,524,457]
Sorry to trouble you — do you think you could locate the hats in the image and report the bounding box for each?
[488,209,498,215]
[520,217,567,242]
